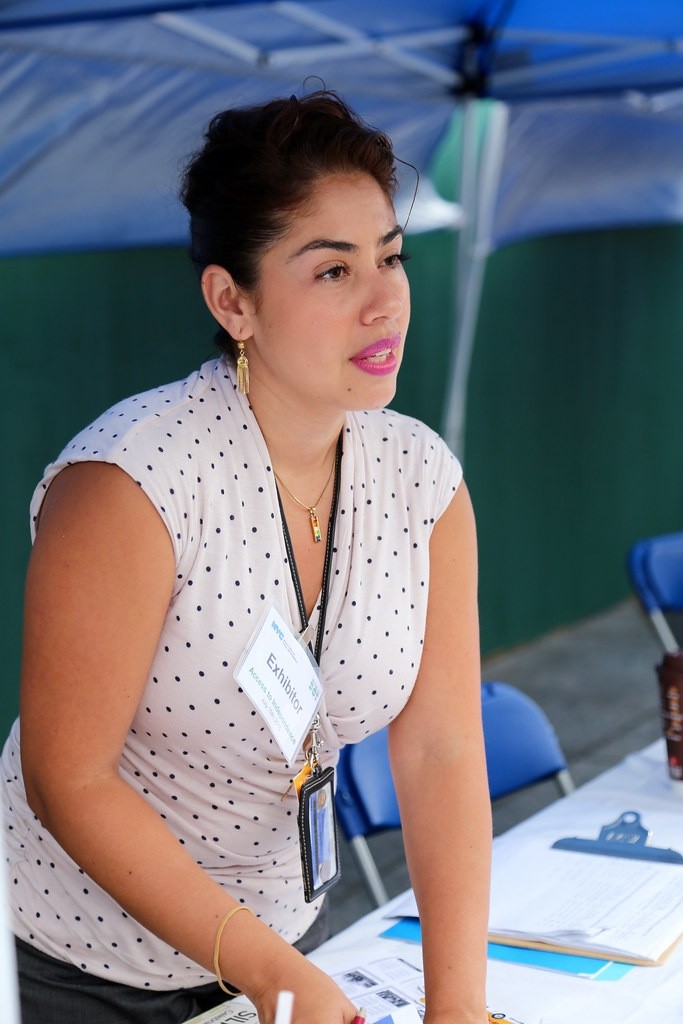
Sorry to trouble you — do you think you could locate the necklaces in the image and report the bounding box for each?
[274,459,335,541]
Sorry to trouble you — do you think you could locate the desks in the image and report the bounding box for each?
[181,739,683,1024]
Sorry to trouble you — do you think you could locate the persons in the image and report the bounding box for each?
[0,91,511,1024]
[316,790,328,809]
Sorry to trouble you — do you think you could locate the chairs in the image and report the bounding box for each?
[628,534,683,654]
[334,679,576,909]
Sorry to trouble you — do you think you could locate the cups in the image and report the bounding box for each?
[655,651,683,780]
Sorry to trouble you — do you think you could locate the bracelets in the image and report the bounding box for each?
[214,906,256,996]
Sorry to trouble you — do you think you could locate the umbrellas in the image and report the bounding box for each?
[0,0,683,471]
